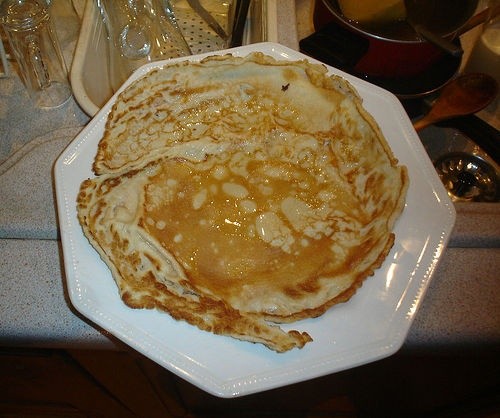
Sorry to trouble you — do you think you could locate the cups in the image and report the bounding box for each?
[475,29,500,82]
[0,0,72,108]
[97,0,193,91]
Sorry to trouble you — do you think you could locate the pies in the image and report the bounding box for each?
[76,50,410,352]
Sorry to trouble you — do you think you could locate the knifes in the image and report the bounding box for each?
[182,0,232,43]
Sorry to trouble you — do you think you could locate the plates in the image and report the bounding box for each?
[53,41,458,398]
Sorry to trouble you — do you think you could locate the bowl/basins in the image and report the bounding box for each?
[299,31,464,127]
[313,0,480,52]
